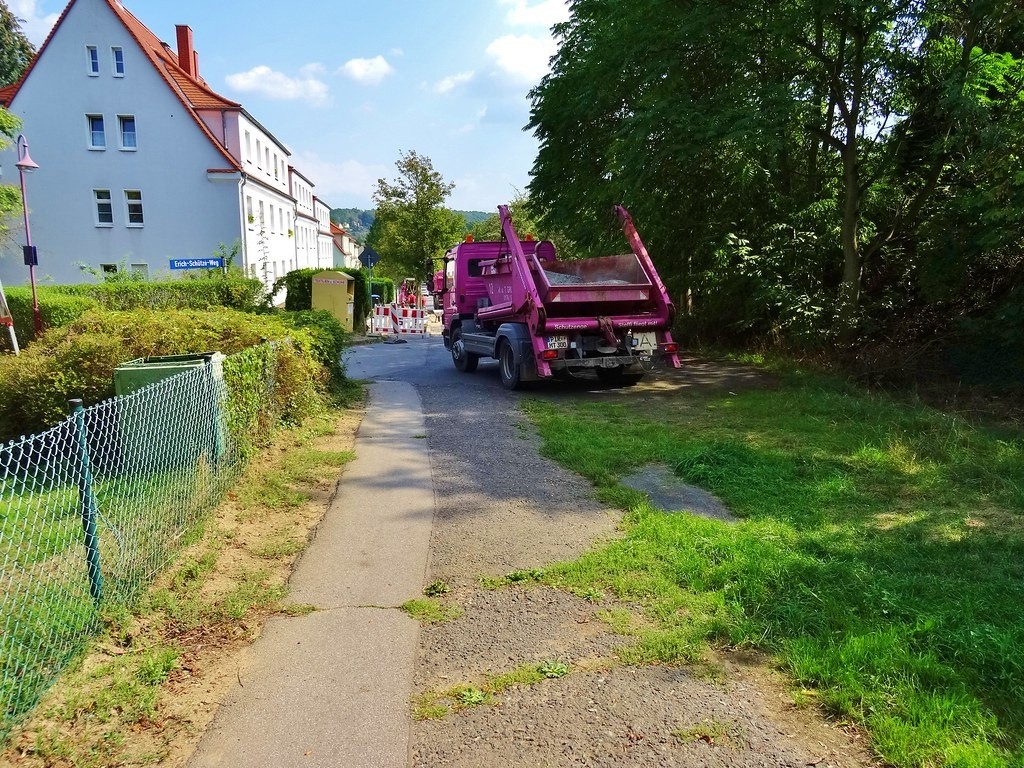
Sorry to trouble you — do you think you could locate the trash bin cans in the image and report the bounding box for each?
[110,347,235,479]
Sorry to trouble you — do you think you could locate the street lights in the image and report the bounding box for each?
[14,133,43,341]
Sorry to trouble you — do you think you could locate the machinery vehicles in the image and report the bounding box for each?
[425,203,682,392]
